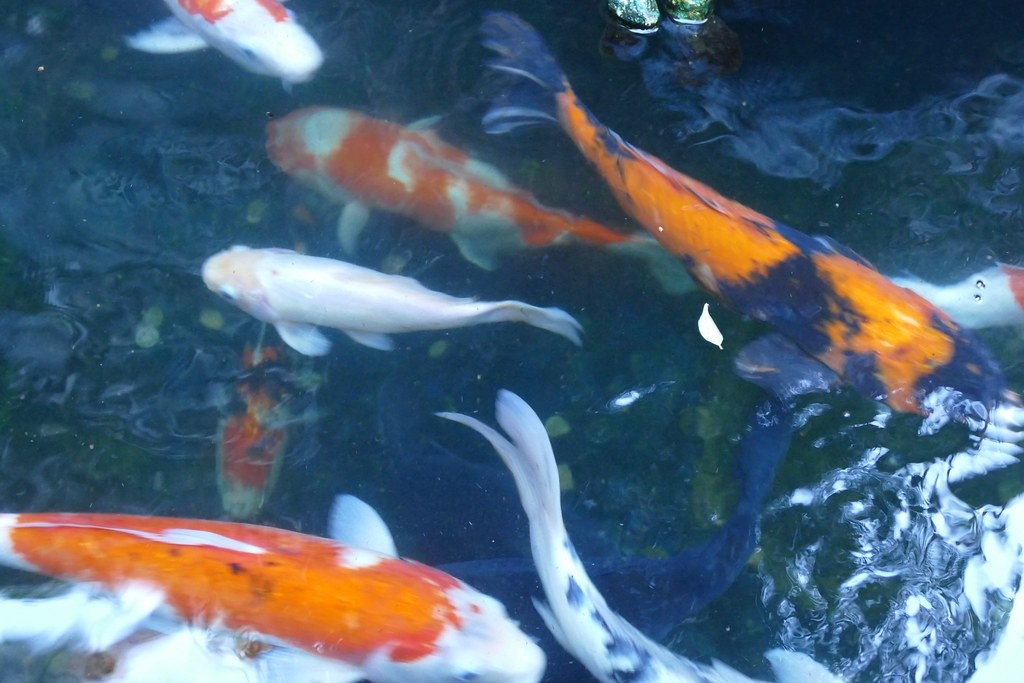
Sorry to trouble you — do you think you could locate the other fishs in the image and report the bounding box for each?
[473,9,1023,448]
[433,386,848,683]
[124,1,326,84]
[2,492,546,683]
[263,103,665,276]
[213,329,298,522]
[202,241,587,356]
